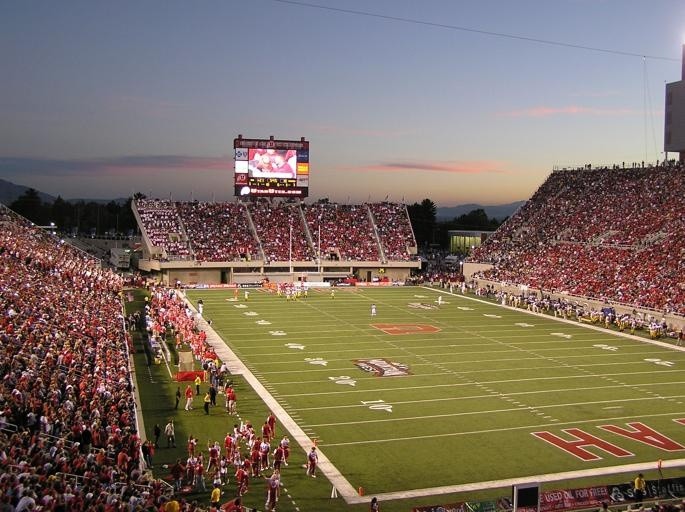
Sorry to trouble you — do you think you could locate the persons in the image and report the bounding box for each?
[0,145,684,512]
